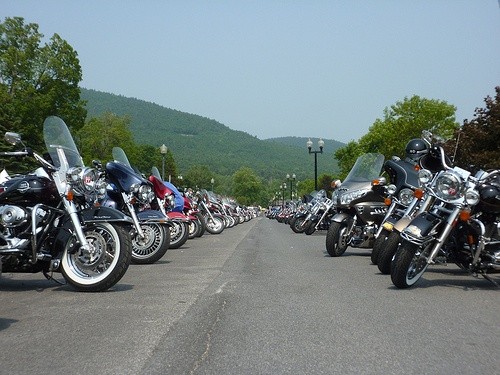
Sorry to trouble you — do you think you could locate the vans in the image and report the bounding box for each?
[247,206,258,211]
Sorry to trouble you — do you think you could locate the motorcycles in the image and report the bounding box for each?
[0,113,260,293]
[265,129,500,290]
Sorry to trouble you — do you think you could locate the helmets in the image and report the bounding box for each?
[406,139,427,161]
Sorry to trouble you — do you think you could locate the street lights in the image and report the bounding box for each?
[267,196,278,205]
[275,192,282,205]
[306,136,325,190]
[211,177,215,192]
[285,172,296,202]
[279,184,287,205]
[159,143,168,181]
